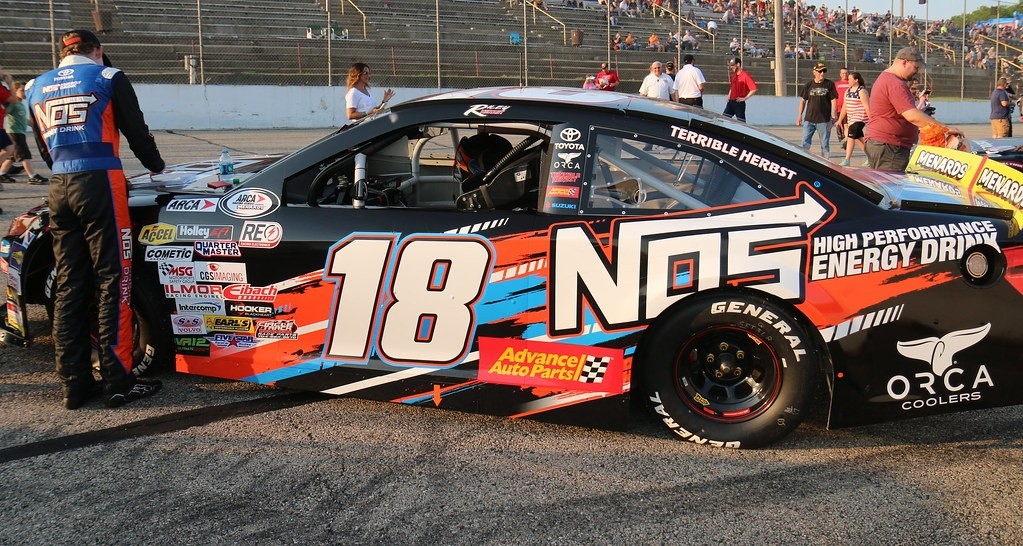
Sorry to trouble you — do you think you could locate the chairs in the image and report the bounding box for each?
[455,146,543,211]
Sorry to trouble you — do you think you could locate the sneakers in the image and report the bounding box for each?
[105,376,162,407]
[0,174,16,183]
[65,379,111,409]
[28,174,49,184]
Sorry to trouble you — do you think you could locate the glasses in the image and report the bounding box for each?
[362,71,370,76]
[602,66,607,69]
[815,68,827,74]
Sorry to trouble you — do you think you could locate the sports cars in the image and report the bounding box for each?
[941,130,1023,175]
[0,87,1023,449]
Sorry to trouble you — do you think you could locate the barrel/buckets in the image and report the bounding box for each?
[91,9,113,31]
[510,33,519,44]
[571,30,585,44]
[866,51,875,61]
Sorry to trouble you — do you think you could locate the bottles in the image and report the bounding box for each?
[219,148,234,190]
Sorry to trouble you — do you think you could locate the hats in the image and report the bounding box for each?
[666,62,674,70]
[59,30,112,68]
[684,55,694,64]
[813,63,827,71]
[729,57,741,66]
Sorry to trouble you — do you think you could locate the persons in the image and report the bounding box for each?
[796,63,870,166]
[639,54,706,109]
[23,30,166,411]
[583,62,620,91]
[989,73,1023,138]
[0,70,50,185]
[345,63,395,123]
[722,58,758,123]
[525,0,1023,70]
[862,46,965,171]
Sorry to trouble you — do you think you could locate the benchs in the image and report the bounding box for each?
[0,1,1022,99]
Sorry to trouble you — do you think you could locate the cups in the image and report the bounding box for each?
[925,102,931,106]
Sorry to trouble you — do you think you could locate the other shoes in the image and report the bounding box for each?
[862,160,870,166]
[8,165,25,176]
[839,160,850,166]
